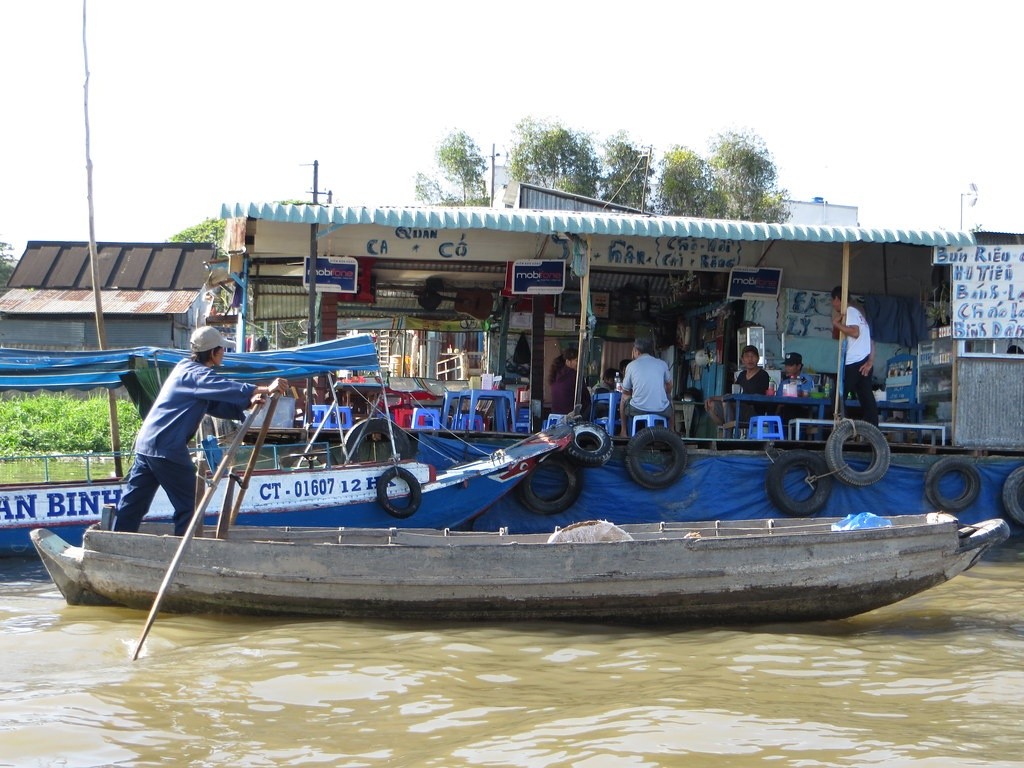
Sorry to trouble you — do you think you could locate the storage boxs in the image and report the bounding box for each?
[238,397,296,428]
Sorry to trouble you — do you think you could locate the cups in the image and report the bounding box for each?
[810,384,818,393]
[732,384,743,394]
[447,348,452,354]
[766,389,775,395]
[347,372,351,380]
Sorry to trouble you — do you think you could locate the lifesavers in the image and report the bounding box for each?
[925,455,981,513]
[515,419,615,516]
[623,426,688,491]
[765,419,891,517]
[377,466,422,519]
[1001,465,1024,528]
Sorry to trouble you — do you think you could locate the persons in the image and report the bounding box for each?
[549,347,592,421]
[592,339,679,436]
[112,326,288,536]
[831,286,880,429]
[706,346,768,438]
[770,352,818,434]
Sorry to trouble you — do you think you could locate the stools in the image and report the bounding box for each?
[589,392,631,437]
[312,389,529,433]
[747,416,784,441]
[546,413,567,429]
[631,414,667,436]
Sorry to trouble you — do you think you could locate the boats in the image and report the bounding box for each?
[29,459,1011,627]
[0,336,583,562]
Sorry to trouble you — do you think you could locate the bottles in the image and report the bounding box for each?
[824,384,830,398]
[920,344,932,365]
[769,378,777,393]
[796,376,802,397]
[613,372,621,391]
[790,375,794,384]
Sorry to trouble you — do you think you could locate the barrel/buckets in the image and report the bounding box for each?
[389,354,402,376]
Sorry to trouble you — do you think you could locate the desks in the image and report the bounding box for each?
[723,393,922,441]
[333,381,388,425]
[673,400,704,438]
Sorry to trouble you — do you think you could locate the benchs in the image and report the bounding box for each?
[788,418,945,446]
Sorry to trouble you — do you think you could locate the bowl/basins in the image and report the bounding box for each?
[810,392,825,399]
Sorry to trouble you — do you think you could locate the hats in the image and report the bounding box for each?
[189,326,236,352]
[780,352,802,365]
[742,345,758,354]
